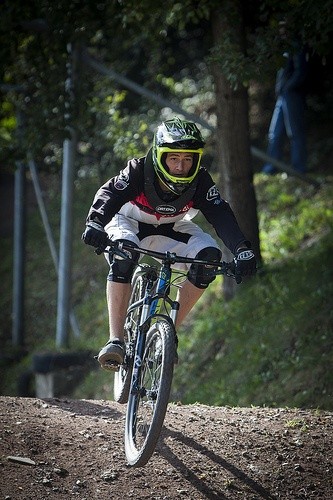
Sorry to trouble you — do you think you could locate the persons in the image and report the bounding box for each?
[261,23,310,174]
[81,115,260,372]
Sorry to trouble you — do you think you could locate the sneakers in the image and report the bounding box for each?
[155,335,178,364]
[99,338,126,372]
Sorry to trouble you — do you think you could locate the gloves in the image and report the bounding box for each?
[235,248,256,284]
[83,222,109,255]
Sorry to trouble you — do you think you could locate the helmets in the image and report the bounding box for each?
[152,116,206,195]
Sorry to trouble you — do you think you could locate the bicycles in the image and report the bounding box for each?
[80,232,260,468]
